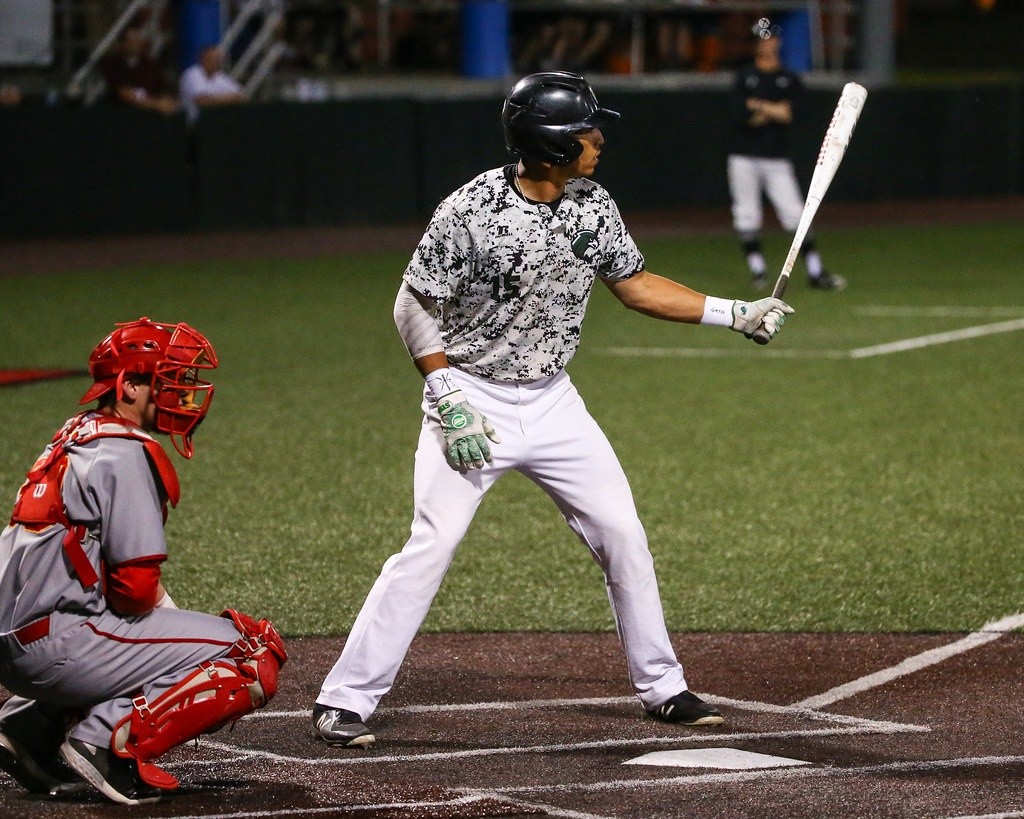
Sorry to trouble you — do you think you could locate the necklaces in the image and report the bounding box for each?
[515,164,528,203]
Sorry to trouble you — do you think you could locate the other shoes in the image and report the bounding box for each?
[751,270,774,292]
[808,269,837,291]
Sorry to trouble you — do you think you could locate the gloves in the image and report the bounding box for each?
[436,391,501,475]
[730,297,795,346]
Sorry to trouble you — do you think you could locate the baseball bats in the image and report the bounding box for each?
[752,81,870,347]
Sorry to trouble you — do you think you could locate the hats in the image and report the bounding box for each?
[751,17,784,41]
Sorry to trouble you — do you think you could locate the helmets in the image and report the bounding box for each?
[76,318,177,402]
[502,68,621,170]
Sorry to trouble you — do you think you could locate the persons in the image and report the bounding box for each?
[727,13,847,292]
[102,0,864,115]
[0,316,288,805]
[310,71,795,749]
[0,74,22,106]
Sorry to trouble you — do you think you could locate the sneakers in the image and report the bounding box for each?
[311,703,378,747]
[644,690,726,729]
[61,736,164,804]
[0,731,57,789]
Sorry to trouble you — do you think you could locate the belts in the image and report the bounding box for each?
[10,615,50,646]
